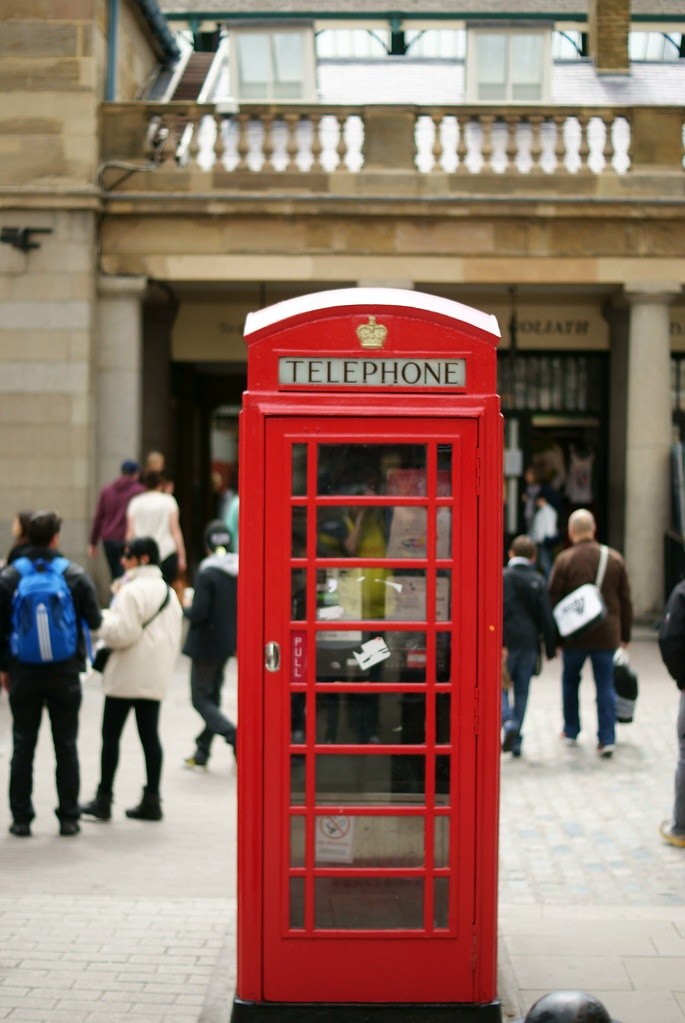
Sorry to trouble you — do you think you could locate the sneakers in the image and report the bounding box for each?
[184,758,207,772]
[661,820,685,848]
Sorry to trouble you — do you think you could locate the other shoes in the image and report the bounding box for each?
[559,732,577,747]
[597,744,614,757]
[10,823,31,837]
[60,820,80,836]
[502,721,522,757]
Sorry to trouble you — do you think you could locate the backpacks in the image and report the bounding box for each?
[10,557,77,664]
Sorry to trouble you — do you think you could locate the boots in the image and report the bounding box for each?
[126,786,162,821]
[79,784,113,819]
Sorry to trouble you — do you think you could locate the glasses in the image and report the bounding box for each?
[122,552,133,561]
[51,509,60,534]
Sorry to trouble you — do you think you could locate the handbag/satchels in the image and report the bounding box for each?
[613,646,638,723]
[532,641,542,675]
[172,570,185,603]
[92,648,111,673]
[553,584,609,638]
[545,538,557,550]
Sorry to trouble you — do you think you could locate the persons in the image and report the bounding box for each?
[0,512,106,836]
[88,451,237,591]
[80,538,183,820]
[180,520,239,773]
[548,509,633,758]
[514,430,601,569]
[0,511,34,567]
[658,579,685,847]
[501,533,557,758]
[291,447,433,752]
